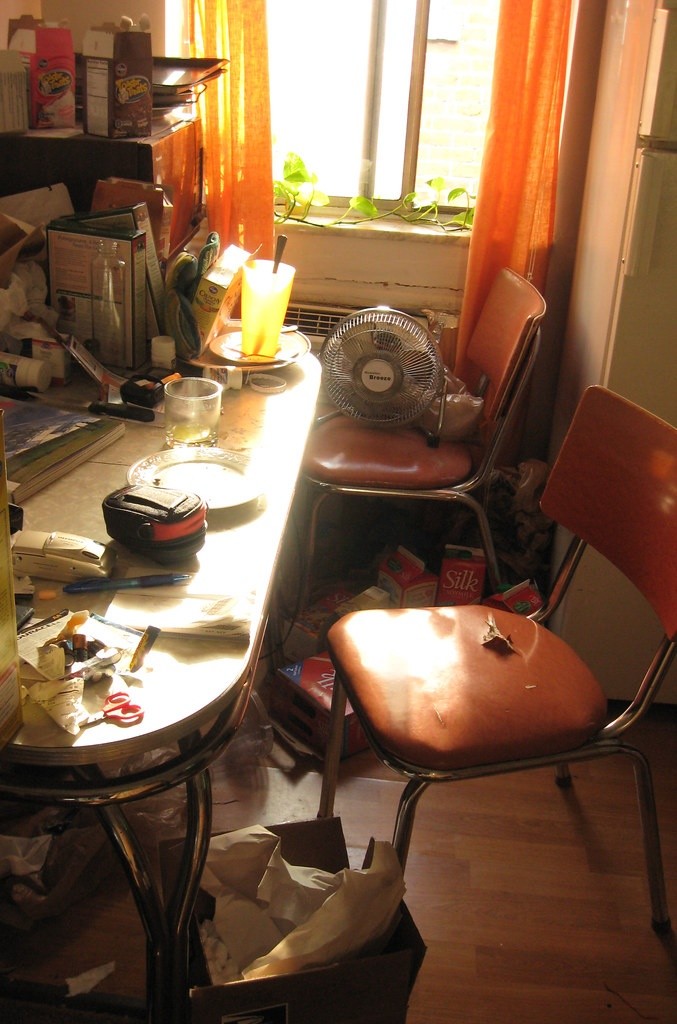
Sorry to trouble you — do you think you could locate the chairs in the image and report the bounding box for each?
[296,268,547,628]
[314,384,677,943]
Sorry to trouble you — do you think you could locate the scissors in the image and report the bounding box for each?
[78,691,144,726]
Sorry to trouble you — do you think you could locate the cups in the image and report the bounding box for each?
[163,377,223,449]
[240,259,296,362]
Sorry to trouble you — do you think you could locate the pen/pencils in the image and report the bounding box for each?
[61,573,193,594]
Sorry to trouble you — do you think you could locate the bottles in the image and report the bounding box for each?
[0,351,52,392]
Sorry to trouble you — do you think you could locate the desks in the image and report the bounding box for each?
[0,321,327,1024]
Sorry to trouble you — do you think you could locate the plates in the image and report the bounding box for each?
[178,325,312,374]
[127,445,263,510]
[208,331,301,366]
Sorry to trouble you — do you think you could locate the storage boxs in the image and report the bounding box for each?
[48,176,174,372]
[269,545,543,760]
[0,49,29,133]
[6,14,77,130]
[0,414,23,750]
[81,22,153,139]
[157,817,428,1024]
[193,242,262,357]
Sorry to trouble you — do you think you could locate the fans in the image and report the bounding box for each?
[314,306,449,448]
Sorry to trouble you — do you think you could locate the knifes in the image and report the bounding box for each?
[27,390,155,422]
